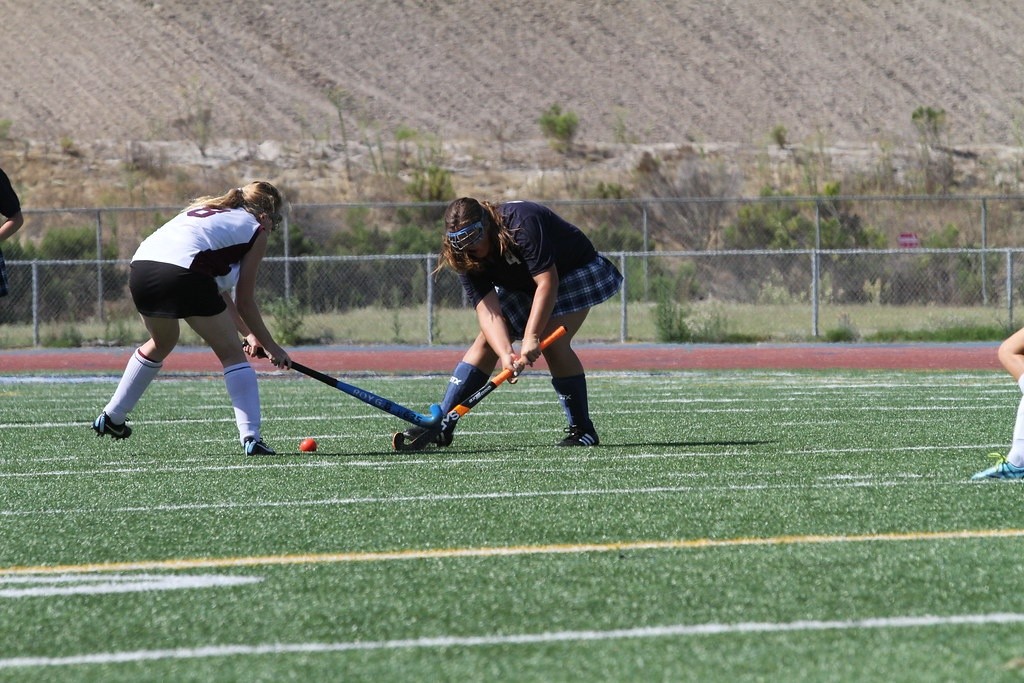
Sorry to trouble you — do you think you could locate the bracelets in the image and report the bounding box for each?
[522,333,538,342]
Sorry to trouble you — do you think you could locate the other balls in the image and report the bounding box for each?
[299,438,317,452]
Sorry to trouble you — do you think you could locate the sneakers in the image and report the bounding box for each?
[90,411,132,441]
[402,424,453,447]
[243,436,276,456]
[557,420,599,447]
[972,451,1024,481]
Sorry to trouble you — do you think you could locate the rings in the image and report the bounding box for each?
[286,359,291,363]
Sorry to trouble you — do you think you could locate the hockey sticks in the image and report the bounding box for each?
[240,339,444,429]
[390,324,570,453]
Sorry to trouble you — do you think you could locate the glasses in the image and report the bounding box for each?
[272,219,280,230]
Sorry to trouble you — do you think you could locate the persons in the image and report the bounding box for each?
[89,180,292,456]
[0,168,24,298]
[402,197,624,446]
[971,328,1024,481]
